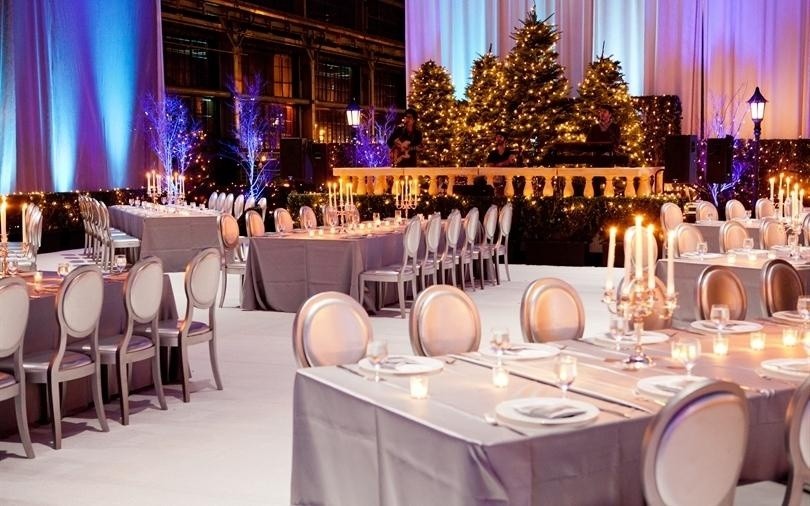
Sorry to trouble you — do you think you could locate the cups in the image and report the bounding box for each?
[367,338,388,384]
[707,212,714,227]
[327,206,338,227]
[710,303,730,336]
[190,202,196,209]
[797,294,810,329]
[675,336,700,384]
[317,229,324,238]
[744,210,752,225]
[553,353,578,398]
[747,253,758,268]
[373,212,380,222]
[749,331,767,352]
[161,197,167,205]
[129,198,134,208]
[696,242,708,264]
[490,326,509,353]
[788,234,799,255]
[409,376,429,399]
[134,200,140,209]
[712,334,729,356]
[329,224,337,235]
[115,255,127,274]
[727,254,737,266]
[142,201,147,212]
[308,228,315,239]
[791,244,803,264]
[767,250,777,260]
[358,223,366,230]
[743,238,755,257]
[395,210,401,220]
[345,205,355,230]
[782,326,799,349]
[383,218,390,228]
[492,360,509,389]
[57,263,70,280]
[199,204,205,210]
[5,259,18,277]
[488,326,510,366]
[366,221,373,230]
[33,271,43,292]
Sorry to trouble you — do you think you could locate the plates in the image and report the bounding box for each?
[477,342,562,361]
[594,330,670,346]
[357,356,445,375]
[494,396,600,425]
[687,320,764,334]
[759,358,810,379]
[772,310,810,323]
[635,374,710,399]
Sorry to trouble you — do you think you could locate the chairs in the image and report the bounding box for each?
[87,196,114,259]
[803,214,810,246]
[223,193,234,216]
[755,198,775,219]
[698,266,747,321]
[382,212,441,308]
[125,248,223,403]
[208,191,217,209]
[244,210,265,237]
[238,197,267,261]
[456,204,498,291]
[345,205,360,222]
[293,291,373,367]
[7,201,44,271]
[410,285,482,357]
[322,204,339,226]
[409,208,462,288]
[519,278,585,344]
[675,223,703,257]
[660,202,683,257]
[719,220,748,253]
[428,207,479,292]
[475,203,513,282]
[1,265,110,449]
[734,375,810,506]
[725,199,746,221]
[215,192,225,211]
[695,200,719,223]
[0,277,34,458]
[760,218,788,249]
[760,259,805,320]
[216,213,247,307]
[784,200,805,216]
[300,206,318,229]
[91,198,133,271]
[274,208,293,232]
[84,196,96,260]
[357,216,422,318]
[616,272,672,331]
[64,255,168,426]
[98,201,140,273]
[78,194,88,255]
[242,195,255,216]
[639,380,750,506]
[233,194,243,218]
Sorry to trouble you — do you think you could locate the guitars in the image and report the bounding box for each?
[495,154,517,167]
[389,140,434,165]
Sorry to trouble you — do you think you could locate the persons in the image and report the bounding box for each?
[387,108,422,167]
[486,132,515,167]
[586,104,621,168]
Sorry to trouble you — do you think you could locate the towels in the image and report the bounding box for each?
[516,400,586,420]
[380,354,420,370]
[655,377,695,393]
[699,319,749,333]
[605,331,654,341]
[778,361,810,374]
[782,308,803,320]
[496,342,530,353]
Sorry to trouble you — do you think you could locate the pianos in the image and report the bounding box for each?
[541,142,629,166]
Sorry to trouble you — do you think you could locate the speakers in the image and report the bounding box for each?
[706,137,733,184]
[280,139,303,177]
[309,143,327,177]
[662,134,698,184]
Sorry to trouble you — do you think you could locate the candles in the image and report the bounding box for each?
[182,176,185,194]
[727,254,734,262]
[328,183,332,207]
[748,251,755,261]
[34,271,41,282]
[647,226,657,291]
[769,177,774,200]
[359,223,362,229]
[794,182,798,216]
[748,333,760,348]
[395,180,399,203]
[606,232,615,290]
[159,175,162,192]
[367,222,370,228]
[791,191,794,219]
[713,338,726,355]
[346,183,349,203]
[179,176,181,193]
[785,177,790,198]
[785,330,797,348]
[667,231,674,299]
[401,181,404,204]
[156,174,159,191]
[317,228,321,235]
[333,183,337,207]
[167,176,169,195]
[308,231,313,235]
[409,182,412,202]
[623,236,631,297]
[684,339,696,361]
[800,189,804,213]
[779,173,783,189]
[330,227,335,233]
[405,176,408,201]
[769,251,777,259]
[778,189,783,213]
[415,181,417,202]
[493,364,507,386]
[634,225,643,280]
[411,375,427,398]
[174,173,178,185]
[350,184,353,204]
[339,179,342,205]
[147,171,151,193]
[1,200,6,241]
[152,170,155,188]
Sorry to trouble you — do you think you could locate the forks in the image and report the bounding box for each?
[753,369,801,387]
[483,410,528,438]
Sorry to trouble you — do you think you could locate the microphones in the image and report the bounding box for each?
[397,122,407,128]
